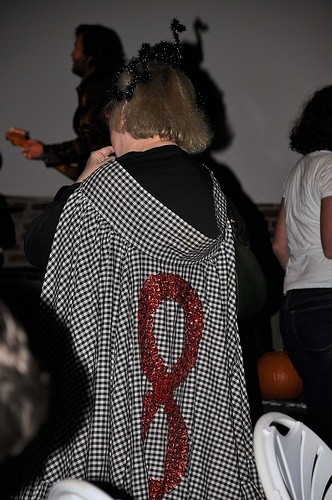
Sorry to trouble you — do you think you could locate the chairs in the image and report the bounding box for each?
[254,412,332,500]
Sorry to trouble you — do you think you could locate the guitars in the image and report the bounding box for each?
[5,126,79,181]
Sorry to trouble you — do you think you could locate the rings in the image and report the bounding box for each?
[24,148,29,153]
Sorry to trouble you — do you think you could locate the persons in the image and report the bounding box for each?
[19,23,127,173]
[271,84,332,449]
[7,58,268,500]
[0,272,92,500]
